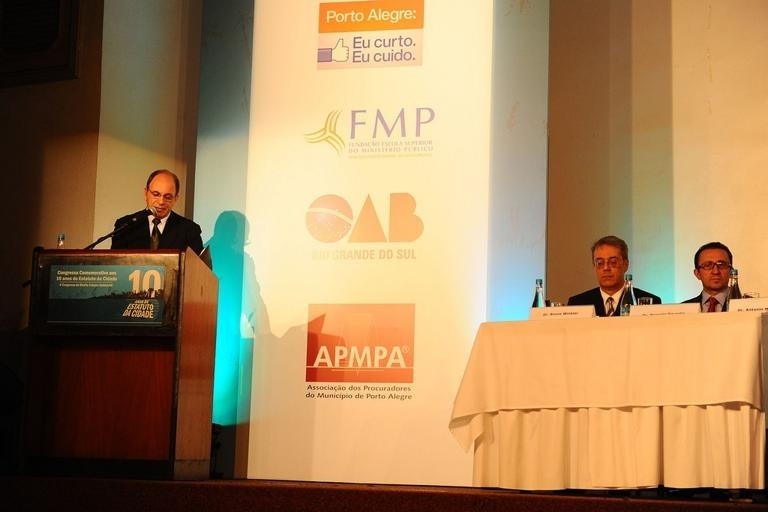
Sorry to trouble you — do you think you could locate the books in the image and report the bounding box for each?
[193,244,214,272]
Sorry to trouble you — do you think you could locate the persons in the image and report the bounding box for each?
[110,166,204,258]
[565,234,662,316]
[678,240,755,314]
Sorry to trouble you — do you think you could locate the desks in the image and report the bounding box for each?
[446,310,768,505]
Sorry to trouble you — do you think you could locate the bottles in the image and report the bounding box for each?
[533,279,546,309]
[620,274,637,315]
[726,269,742,312]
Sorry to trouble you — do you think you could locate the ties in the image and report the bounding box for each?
[707,297,719,313]
[147,215,164,251]
[604,296,616,317]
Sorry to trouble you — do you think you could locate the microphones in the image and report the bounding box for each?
[132,207,156,221]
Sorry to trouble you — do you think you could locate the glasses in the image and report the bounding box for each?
[144,185,176,202]
[695,261,731,271]
[593,257,625,267]
